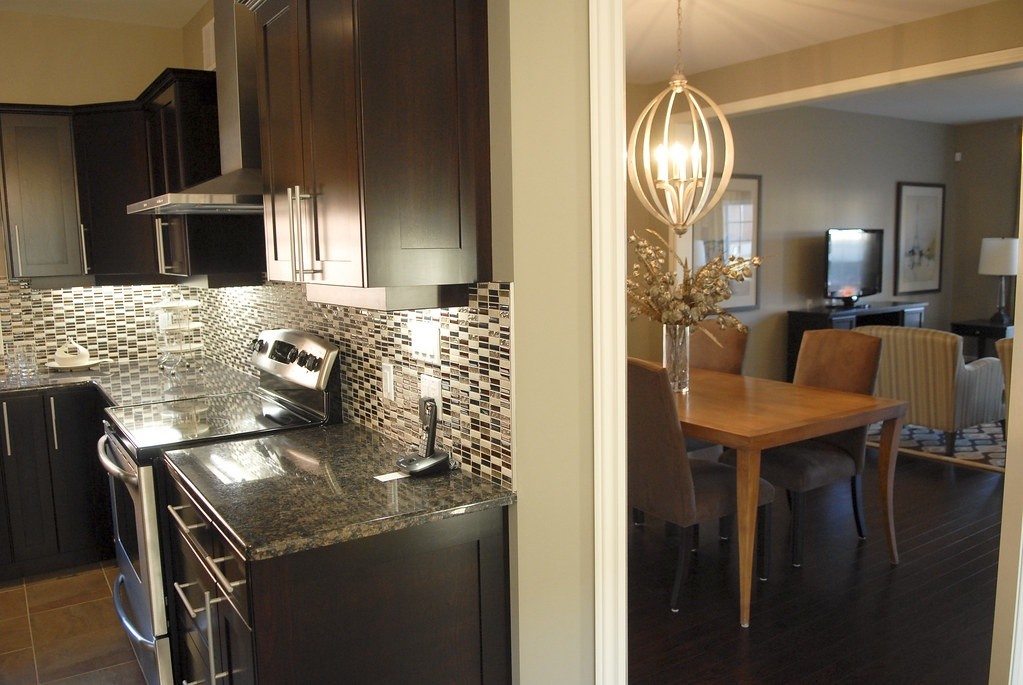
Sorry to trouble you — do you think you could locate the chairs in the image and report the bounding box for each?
[719,328,882,568]
[850,324,1006,456]
[684,317,751,450]
[624,358,776,613]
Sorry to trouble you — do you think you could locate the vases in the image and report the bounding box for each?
[661,323,689,393]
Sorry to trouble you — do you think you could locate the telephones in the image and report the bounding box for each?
[394,397,452,476]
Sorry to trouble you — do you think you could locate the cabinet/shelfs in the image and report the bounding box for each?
[0,389,115,586]
[163,467,512,685]
[237,0,494,312]
[788,301,928,383]
[0,67,227,288]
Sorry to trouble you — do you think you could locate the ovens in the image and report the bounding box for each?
[96,425,323,685]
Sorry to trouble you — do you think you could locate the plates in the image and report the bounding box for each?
[156,343,203,353]
[45,358,113,372]
[144,300,200,308]
[160,321,205,331]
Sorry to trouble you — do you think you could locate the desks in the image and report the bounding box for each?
[638,358,910,629]
[951,318,1015,360]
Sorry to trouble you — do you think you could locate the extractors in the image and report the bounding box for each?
[126,0,264,217]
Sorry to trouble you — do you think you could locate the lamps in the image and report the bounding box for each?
[977,236,1019,325]
[627,1,735,235]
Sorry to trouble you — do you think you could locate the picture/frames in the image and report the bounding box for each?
[893,182,946,295]
[689,175,761,311]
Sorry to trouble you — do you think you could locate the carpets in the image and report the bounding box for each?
[862,421,1006,472]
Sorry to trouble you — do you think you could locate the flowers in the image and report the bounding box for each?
[623,226,764,381]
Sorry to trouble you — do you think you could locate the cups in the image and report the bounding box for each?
[0,352,39,390]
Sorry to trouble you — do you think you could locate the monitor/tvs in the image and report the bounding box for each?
[824,229,884,308]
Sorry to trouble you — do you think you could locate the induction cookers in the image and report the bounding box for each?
[102,327,343,468]
